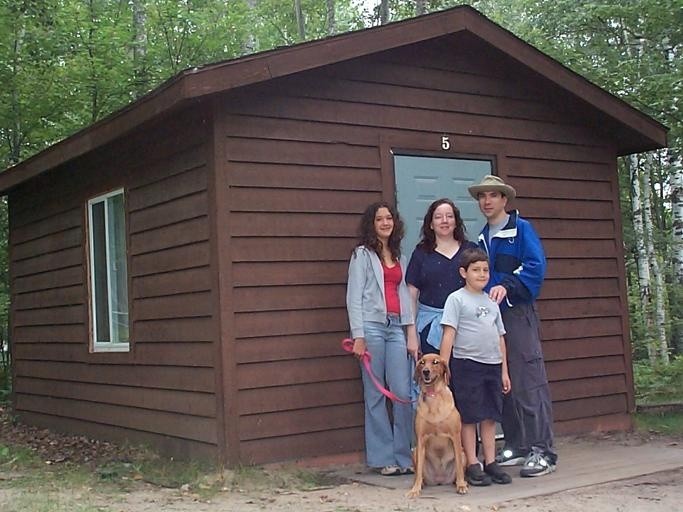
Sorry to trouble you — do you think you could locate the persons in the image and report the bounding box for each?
[345,199,417,476]
[438,246,514,486]
[466,174,558,478]
[403,197,486,457]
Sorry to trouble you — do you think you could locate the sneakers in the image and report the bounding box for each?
[483,459,512,484]
[520,446,558,477]
[464,463,492,486]
[372,465,402,475]
[495,445,531,466]
[400,466,415,474]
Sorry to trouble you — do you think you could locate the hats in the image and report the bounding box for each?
[468,174,517,202]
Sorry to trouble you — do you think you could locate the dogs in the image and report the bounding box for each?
[405,352,469,498]
